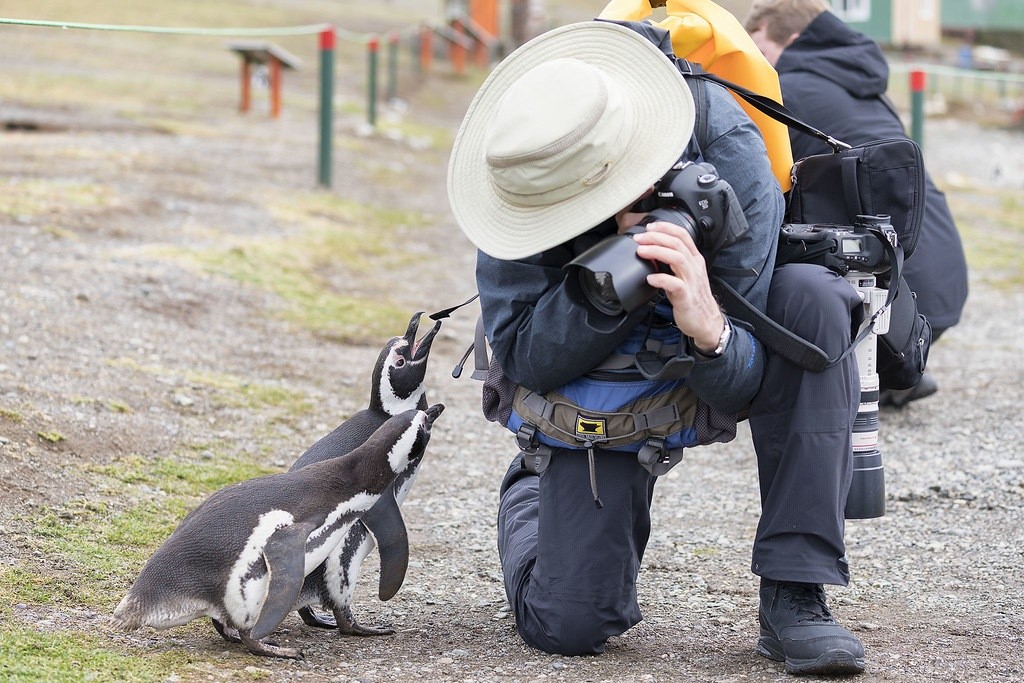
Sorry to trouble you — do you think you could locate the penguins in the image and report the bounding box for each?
[102,309,447,667]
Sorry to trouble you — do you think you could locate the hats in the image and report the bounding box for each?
[444,21,697,261]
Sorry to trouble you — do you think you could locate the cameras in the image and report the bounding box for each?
[775,219,886,519]
[562,159,748,334]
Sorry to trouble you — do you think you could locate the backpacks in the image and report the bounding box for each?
[504,289,702,509]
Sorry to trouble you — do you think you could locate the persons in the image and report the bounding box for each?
[447,18,867,677]
[743,0,968,413]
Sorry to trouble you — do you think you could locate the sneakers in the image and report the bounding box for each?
[499,450,536,500]
[882,369,937,411]
[753,577,865,674]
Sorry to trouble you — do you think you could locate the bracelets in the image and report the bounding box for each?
[688,312,731,359]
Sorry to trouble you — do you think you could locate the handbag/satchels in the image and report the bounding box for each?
[773,136,924,269]
